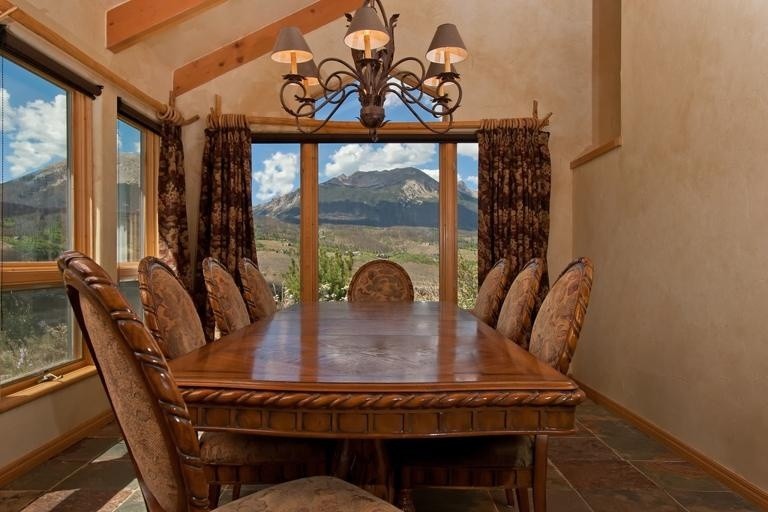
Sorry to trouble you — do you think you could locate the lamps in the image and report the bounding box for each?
[270,0,468,143]
